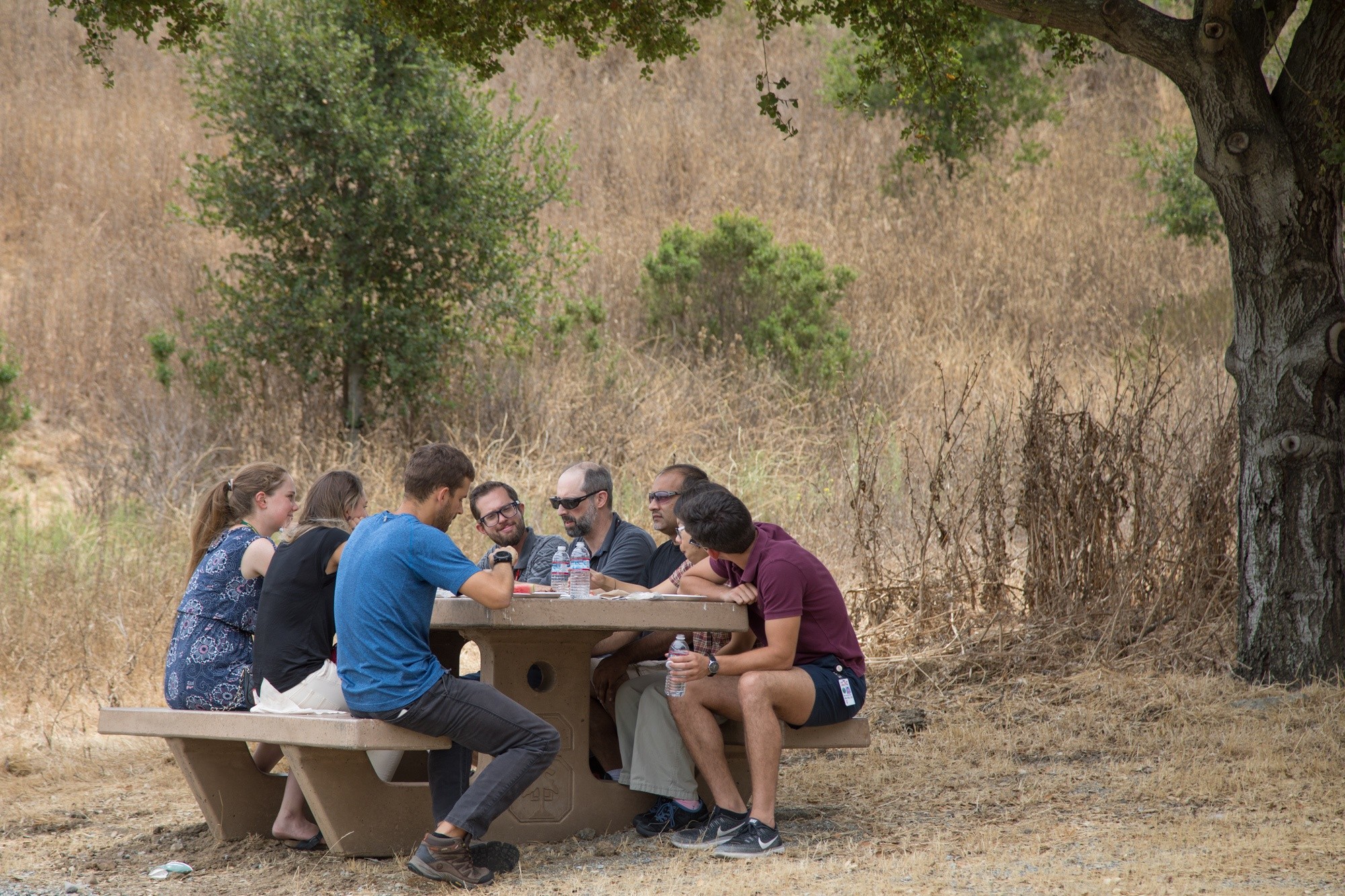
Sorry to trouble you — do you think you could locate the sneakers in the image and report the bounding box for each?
[464,840,521,872]
[408,831,495,887]
[672,806,750,848]
[712,817,785,860]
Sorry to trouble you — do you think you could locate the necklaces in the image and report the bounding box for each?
[237,519,259,535]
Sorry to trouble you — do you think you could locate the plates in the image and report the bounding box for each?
[531,593,561,599]
[662,594,707,601]
[513,593,532,598]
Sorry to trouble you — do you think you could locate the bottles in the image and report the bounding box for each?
[550,546,570,597]
[570,542,590,599]
[665,634,689,697]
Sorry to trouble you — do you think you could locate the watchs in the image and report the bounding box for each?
[492,551,513,564]
[707,654,718,677]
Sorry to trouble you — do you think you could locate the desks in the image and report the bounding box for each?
[332,593,872,861]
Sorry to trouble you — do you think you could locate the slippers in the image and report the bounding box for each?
[285,827,325,850]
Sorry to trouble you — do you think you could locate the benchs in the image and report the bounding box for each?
[97,707,453,857]
[695,710,868,812]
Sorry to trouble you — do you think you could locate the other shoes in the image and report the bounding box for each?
[633,795,707,836]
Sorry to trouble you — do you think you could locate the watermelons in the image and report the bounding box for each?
[514,584,535,594]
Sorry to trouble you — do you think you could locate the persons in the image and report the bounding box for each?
[251,472,405,780]
[452,461,865,857]
[163,463,330,852]
[334,443,560,888]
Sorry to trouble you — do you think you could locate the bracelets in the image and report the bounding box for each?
[614,578,617,590]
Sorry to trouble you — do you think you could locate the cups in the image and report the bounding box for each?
[149,862,192,878]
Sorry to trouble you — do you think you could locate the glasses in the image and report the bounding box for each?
[476,500,521,528]
[648,490,680,502]
[689,538,707,550]
[549,489,606,510]
